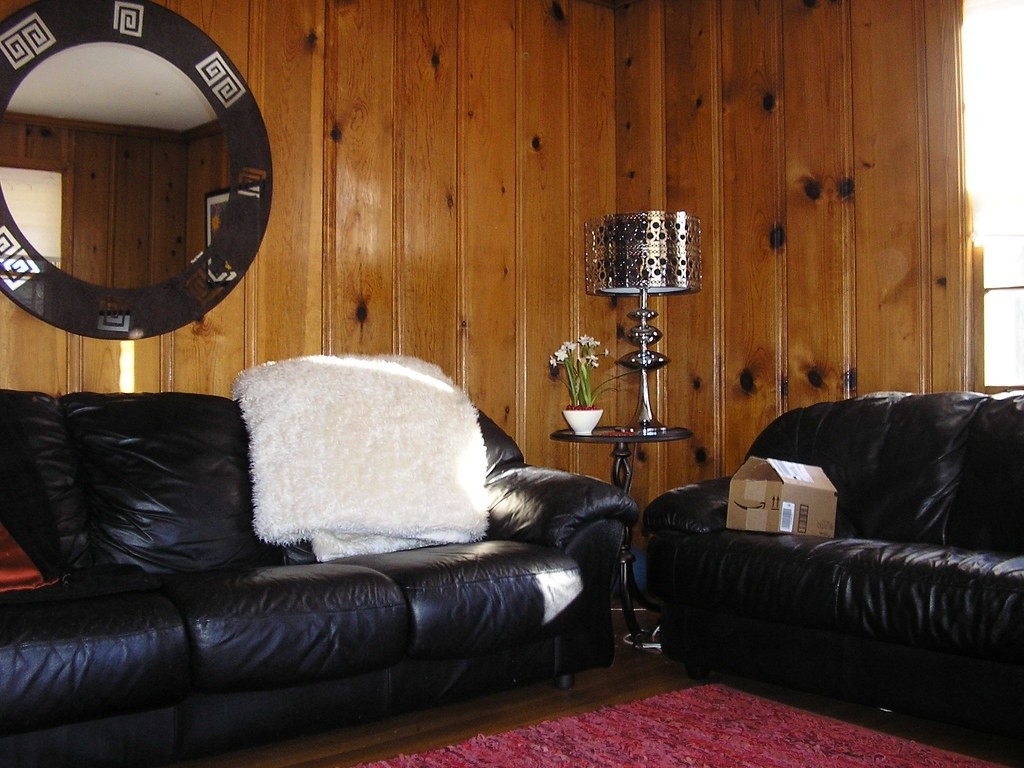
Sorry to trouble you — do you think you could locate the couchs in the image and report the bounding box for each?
[0,354,641,768]
[643,391,1024,741]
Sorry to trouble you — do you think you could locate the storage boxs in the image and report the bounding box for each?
[726,455,838,537]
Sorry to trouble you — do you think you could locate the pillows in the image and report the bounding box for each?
[0,520,59,593]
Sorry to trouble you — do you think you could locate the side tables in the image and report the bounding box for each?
[550,426,693,650]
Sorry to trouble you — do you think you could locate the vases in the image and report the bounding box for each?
[562,408,604,436]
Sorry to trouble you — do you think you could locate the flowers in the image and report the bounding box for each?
[549,334,641,407]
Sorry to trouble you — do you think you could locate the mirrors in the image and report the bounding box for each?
[0,0,273,340]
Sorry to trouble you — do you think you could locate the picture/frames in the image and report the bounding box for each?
[205,181,263,286]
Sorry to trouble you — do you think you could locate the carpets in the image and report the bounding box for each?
[349,683,1012,768]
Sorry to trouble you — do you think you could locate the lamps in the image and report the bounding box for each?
[584,210,703,435]
